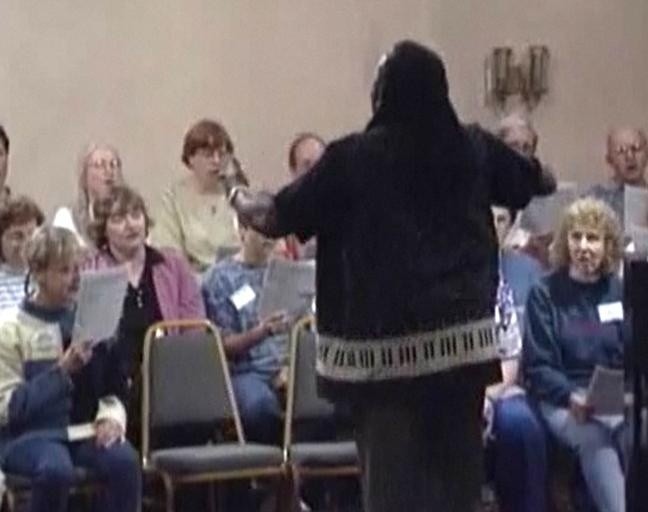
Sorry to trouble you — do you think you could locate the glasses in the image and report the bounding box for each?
[196,150,232,163]
[613,143,642,153]
[89,159,120,170]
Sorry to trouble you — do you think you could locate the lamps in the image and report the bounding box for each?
[483,46,551,113]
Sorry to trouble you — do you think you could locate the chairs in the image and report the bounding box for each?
[3,459,142,512]
[142,319,289,512]
[283,315,361,512]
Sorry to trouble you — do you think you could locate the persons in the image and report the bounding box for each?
[0,120,327,510]
[218,41,540,511]
[479,117,648,510]
